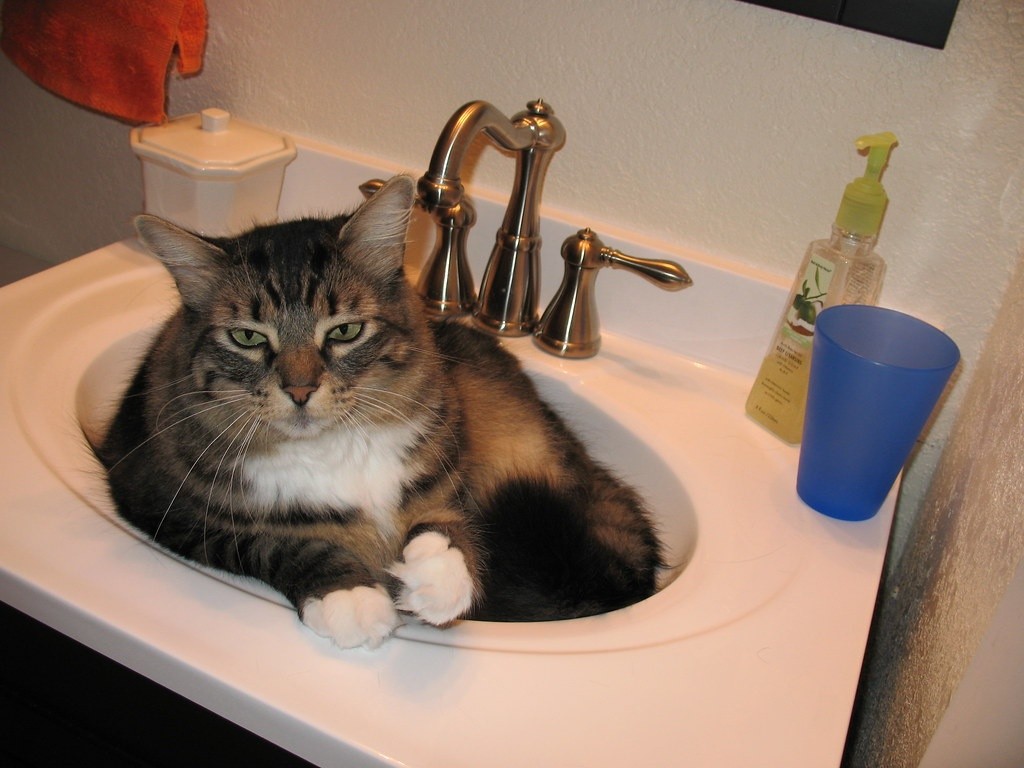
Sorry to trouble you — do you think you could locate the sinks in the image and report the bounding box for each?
[76,315,701,625]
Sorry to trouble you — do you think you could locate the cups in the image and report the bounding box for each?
[134,110,299,236]
[795,304,962,522]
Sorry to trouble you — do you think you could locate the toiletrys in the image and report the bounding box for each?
[744,131,900,446]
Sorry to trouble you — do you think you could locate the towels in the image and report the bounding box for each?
[0,0,210,127]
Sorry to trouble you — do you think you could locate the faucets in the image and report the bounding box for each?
[416,96,568,339]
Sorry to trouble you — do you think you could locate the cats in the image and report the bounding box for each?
[100,175,659,647]
